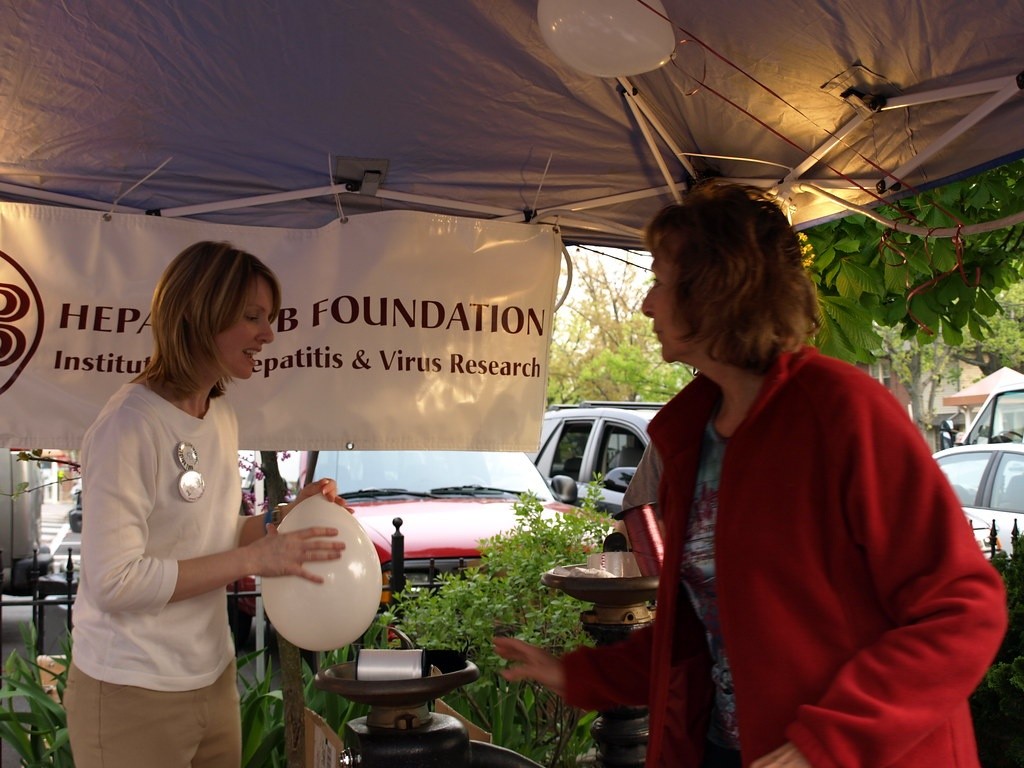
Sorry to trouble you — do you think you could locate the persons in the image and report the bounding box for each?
[491,185,1008,768]
[65,240,355,768]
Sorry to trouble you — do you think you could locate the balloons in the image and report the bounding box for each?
[261,490,383,650]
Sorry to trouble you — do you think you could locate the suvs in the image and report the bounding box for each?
[67,400,669,657]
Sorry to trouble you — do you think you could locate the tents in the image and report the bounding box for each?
[0,0,1023,455]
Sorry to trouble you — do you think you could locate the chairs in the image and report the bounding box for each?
[999,475,1024,510]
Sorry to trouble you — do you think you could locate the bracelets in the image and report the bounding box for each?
[263,509,272,533]
[272,509,280,524]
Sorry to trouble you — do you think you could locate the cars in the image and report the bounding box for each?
[930,443,1024,562]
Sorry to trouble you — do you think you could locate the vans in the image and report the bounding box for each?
[939,382,1024,462]
